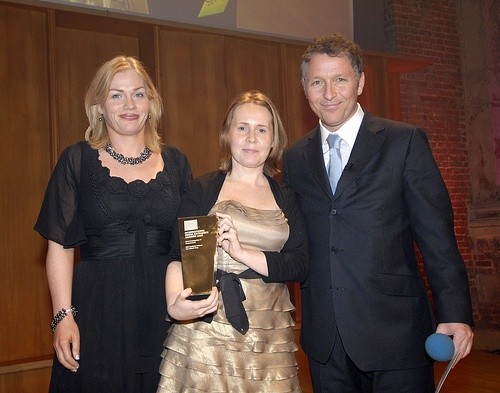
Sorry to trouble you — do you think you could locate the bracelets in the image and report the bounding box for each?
[50,304,78,333]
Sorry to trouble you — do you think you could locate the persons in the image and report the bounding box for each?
[157,89,312,393]
[45,55,195,393]
[282,32,476,393]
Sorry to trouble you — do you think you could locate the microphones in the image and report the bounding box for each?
[425,333,456,362]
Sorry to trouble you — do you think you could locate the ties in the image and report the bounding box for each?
[326,134,342,195]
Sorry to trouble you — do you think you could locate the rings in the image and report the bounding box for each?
[227,225,232,232]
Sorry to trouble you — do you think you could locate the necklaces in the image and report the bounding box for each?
[101,142,153,166]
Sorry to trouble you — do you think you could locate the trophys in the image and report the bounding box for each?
[178,215,217,301]
